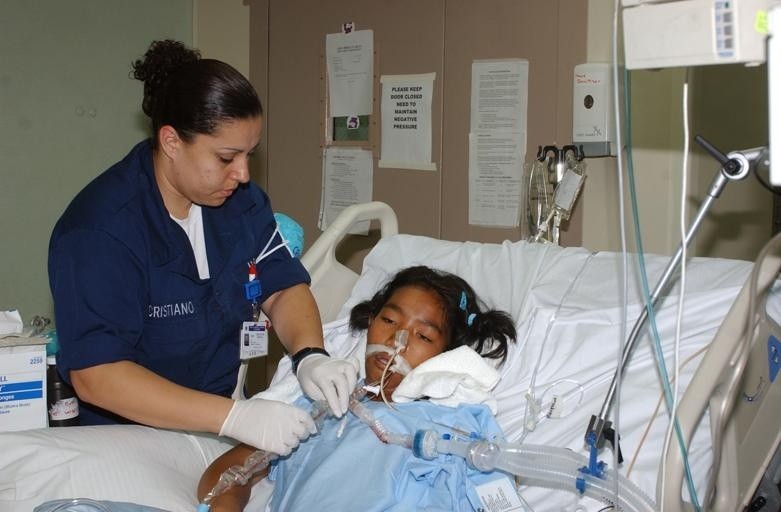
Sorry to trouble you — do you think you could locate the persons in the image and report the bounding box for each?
[199,267,519,512]
[48,39,361,457]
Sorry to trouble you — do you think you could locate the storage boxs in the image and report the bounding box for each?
[1,336,52,432]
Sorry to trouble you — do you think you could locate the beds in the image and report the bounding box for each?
[0,200,781,512]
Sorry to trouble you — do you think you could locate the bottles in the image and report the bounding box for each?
[45,355,81,428]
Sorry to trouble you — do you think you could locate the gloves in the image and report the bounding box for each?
[218,399,317,456]
[296,354,360,419]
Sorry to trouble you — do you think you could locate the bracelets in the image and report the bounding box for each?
[291,347,330,376]
